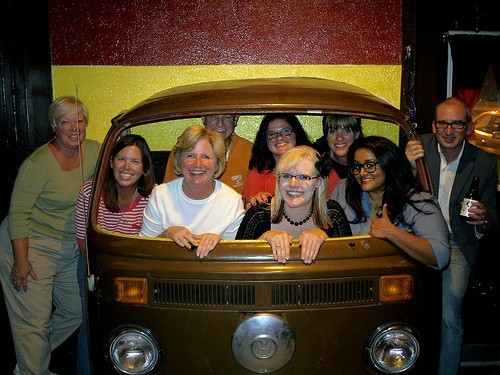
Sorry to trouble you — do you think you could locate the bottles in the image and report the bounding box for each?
[458,176,480,221]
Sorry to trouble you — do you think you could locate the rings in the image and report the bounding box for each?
[13,278,16,282]
[479,216,484,221]
[178,235,182,238]
[276,245,280,247]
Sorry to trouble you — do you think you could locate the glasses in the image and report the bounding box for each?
[435,120,467,129]
[278,173,321,183]
[265,127,295,140]
[349,162,379,175]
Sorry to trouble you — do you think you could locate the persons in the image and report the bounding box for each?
[405,96,500,375]
[74,134,160,375]
[0,95,106,375]
[242,114,340,205]
[235,145,353,264]
[162,113,256,198]
[346,135,452,271]
[317,115,365,179]
[139,124,246,259]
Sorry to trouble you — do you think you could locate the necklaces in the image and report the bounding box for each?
[362,211,381,228]
[282,200,314,226]
[115,189,137,223]
